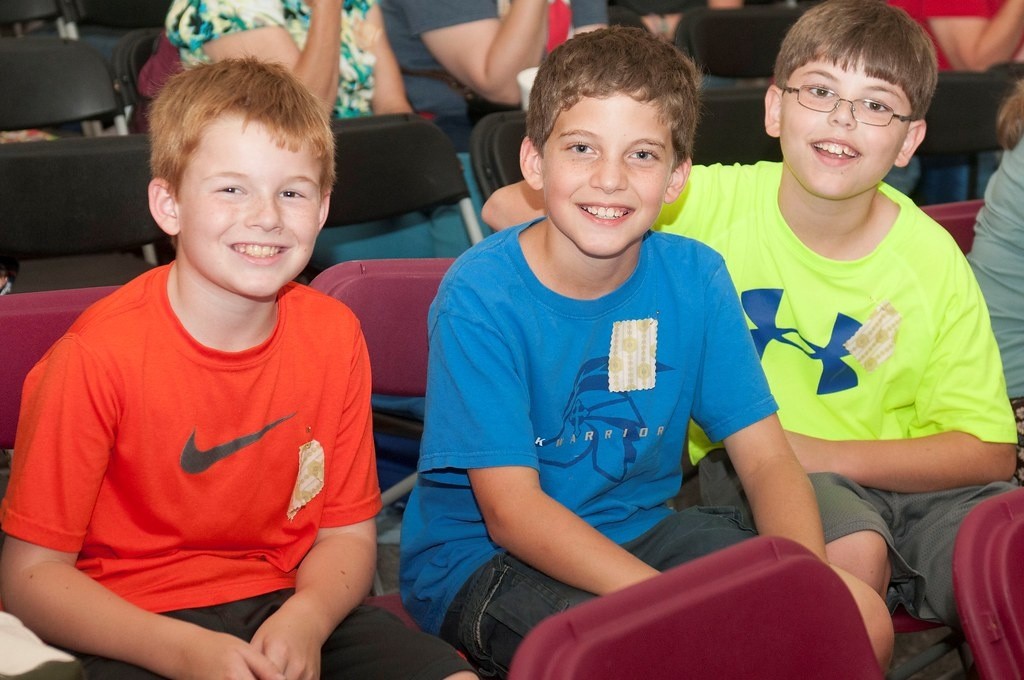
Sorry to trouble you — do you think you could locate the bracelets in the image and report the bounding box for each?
[654,12,668,35]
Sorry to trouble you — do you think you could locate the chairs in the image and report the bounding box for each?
[0,40,119,139]
[306,258,461,634]
[0,135,167,295]
[0,0,69,40]
[953,487,1024,680]
[688,77,786,167]
[62,0,173,40]
[469,111,530,203]
[0,285,124,450]
[321,112,484,246]
[675,8,816,78]
[912,64,1024,153]
[505,535,883,680]
[114,27,164,134]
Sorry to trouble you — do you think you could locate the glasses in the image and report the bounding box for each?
[784,83,914,127]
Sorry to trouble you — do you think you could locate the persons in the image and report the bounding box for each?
[381,1,576,130]
[165,2,412,126]
[1,56,479,680]
[480,2,1023,680]
[400,21,899,680]
[891,1,1024,70]
[611,2,744,79]
[958,74,1024,487]
